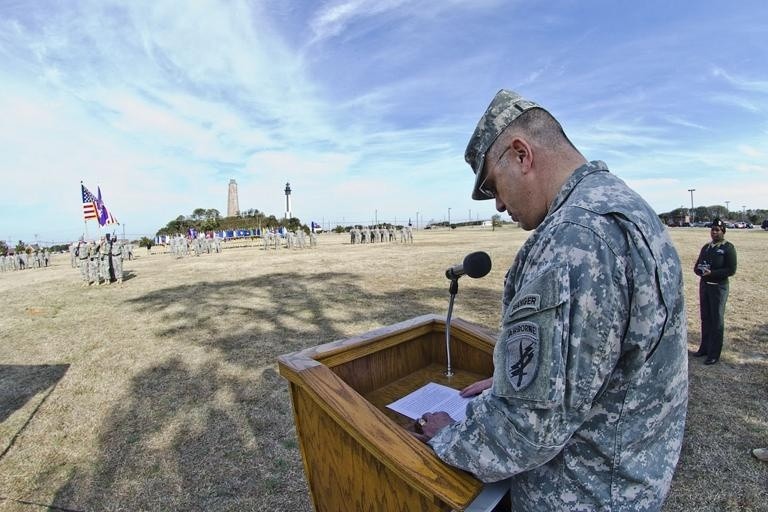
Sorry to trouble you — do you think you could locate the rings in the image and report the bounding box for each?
[417,418,426,426]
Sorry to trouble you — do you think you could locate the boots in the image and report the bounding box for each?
[81,278,122,288]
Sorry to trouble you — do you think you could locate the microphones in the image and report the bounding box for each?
[445,251,492,278]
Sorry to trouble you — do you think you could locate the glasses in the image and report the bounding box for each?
[478,145,511,199]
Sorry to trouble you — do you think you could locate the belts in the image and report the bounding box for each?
[80,253,121,260]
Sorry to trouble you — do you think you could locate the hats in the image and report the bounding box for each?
[78,235,117,244]
[463,87,543,200]
[710,218,726,234]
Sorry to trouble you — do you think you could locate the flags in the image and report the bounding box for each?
[81,181,120,240]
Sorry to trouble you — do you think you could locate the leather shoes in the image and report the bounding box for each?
[704,357,717,365]
[692,348,706,357]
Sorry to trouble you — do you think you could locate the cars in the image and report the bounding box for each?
[688,219,768,231]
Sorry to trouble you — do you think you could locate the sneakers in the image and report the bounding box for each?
[753,445,768,462]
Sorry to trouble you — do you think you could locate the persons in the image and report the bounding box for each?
[350,224,413,245]
[692,218,737,365]
[69,233,134,286]
[264,227,317,250]
[170,232,222,259]
[418,90,690,512]
[0,248,49,271]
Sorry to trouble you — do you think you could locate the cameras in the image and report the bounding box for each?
[695,264,711,275]
